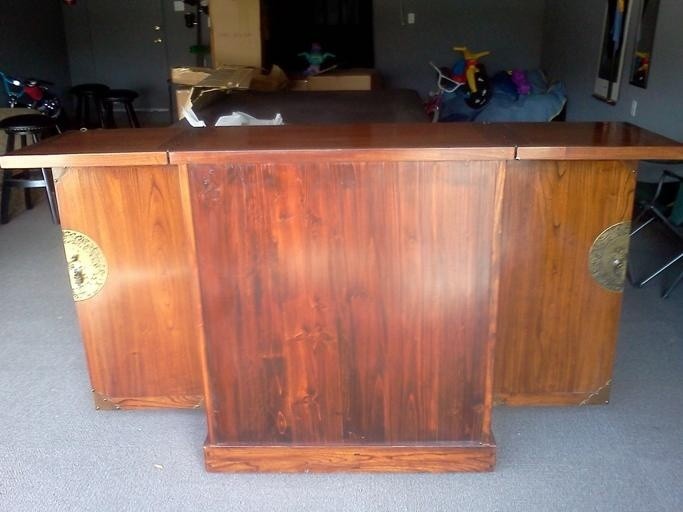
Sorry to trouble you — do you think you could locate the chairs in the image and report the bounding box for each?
[629,168,682,300]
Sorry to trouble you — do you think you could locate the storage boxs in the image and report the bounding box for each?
[170,0,384,119]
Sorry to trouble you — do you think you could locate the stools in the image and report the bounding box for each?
[98,90,142,128]
[69,83,111,129]
[0,115,60,225]
